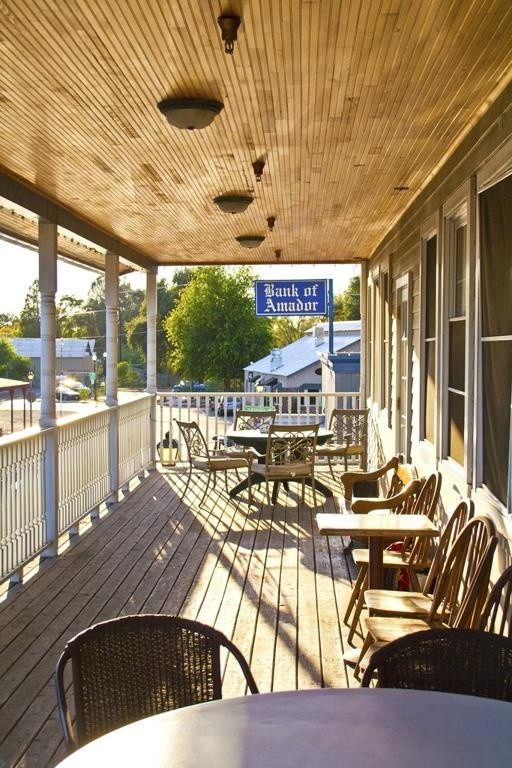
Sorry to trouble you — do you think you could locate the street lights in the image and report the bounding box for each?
[103,351,107,378]
[92,355,96,372]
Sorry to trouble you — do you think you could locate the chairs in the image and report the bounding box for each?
[53,612,258,753]
[354,497,512,701]
[342,453,442,643]
[171,405,369,507]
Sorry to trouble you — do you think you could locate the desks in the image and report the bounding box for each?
[315,511,441,672]
[51,687,512,767]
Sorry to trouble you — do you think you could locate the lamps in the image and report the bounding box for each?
[156,431,180,467]
[156,97,224,132]
[236,236,266,250]
[213,195,255,215]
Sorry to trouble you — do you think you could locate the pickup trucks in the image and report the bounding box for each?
[173,380,206,391]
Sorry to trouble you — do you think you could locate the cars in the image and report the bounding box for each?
[211,396,241,416]
[55,379,91,401]
